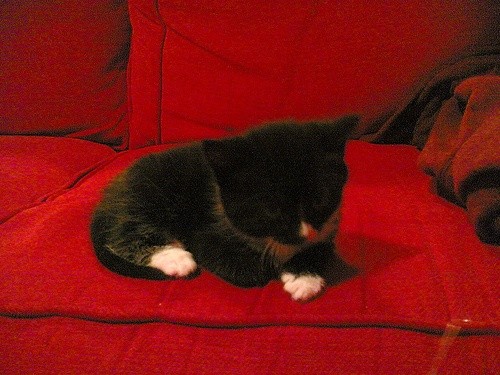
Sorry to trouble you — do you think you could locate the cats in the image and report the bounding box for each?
[88,111,362,304]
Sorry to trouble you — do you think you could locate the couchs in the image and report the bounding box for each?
[1,0,500,375]
